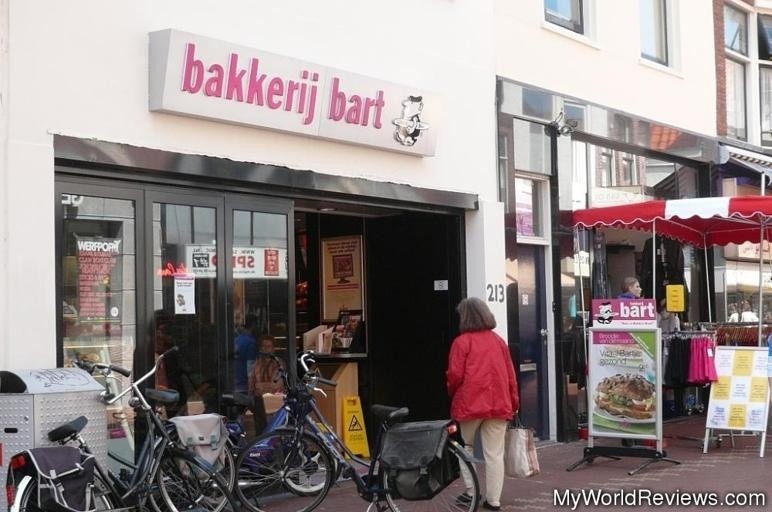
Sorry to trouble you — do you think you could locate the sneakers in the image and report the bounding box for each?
[483,500,500,511]
[457,492,482,503]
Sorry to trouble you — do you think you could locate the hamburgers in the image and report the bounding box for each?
[593,375,657,419]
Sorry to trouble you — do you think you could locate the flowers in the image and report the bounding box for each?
[334,316,360,336]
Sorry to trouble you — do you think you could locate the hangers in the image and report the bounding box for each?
[656,323,772,345]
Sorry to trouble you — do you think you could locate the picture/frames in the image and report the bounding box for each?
[317,233,367,325]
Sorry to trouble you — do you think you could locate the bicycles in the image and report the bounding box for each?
[5,344,347,512]
[233,350,483,512]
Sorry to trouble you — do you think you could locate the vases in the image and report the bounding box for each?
[332,332,357,350]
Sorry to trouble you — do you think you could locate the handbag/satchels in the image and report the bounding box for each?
[168,413,230,479]
[6,447,95,511]
[381,419,463,500]
[505,427,540,478]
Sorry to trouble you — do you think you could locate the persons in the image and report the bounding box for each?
[615,275,644,300]
[725,298,771,329]
[444,292,519,512]
[63,290,296,437]
